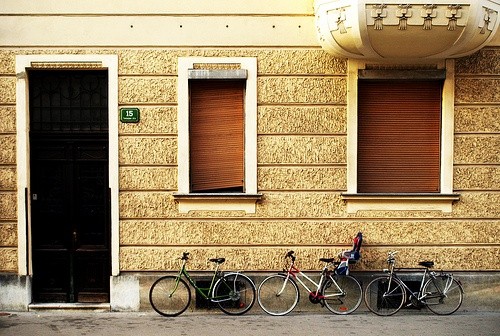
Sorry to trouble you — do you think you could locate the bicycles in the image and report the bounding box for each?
[365,250,464,315]
[258,250,363,315]
[149,252,258,317]
